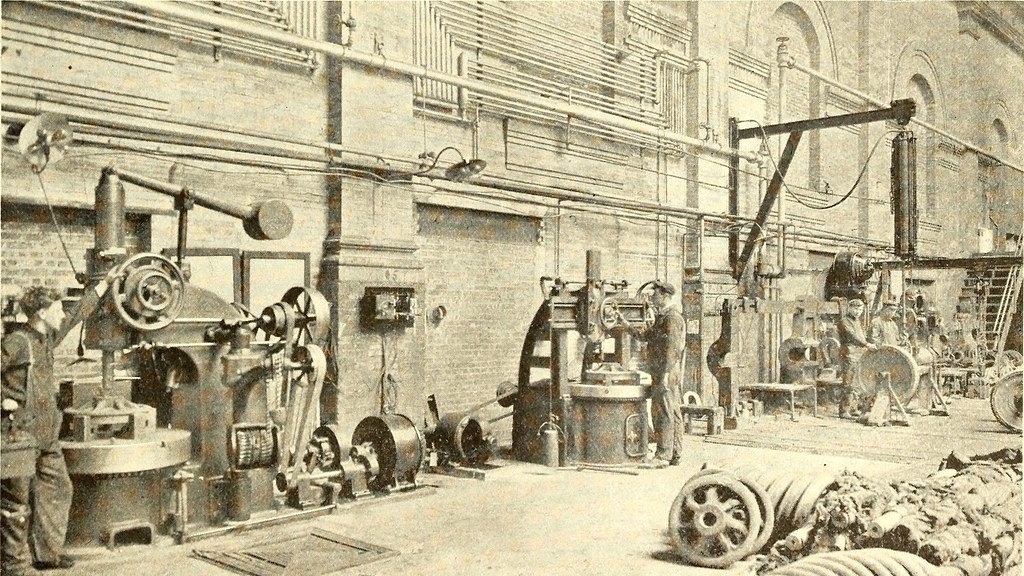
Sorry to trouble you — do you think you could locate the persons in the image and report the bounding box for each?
[0,264,122,576]
[612,281,687,469]
[838,300,878,420]
[867,299,899,349]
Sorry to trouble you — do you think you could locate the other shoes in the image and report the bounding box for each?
[34,554,73,570]
[849,409,861,416]
[839,411,856,420]
[638,455,680,469]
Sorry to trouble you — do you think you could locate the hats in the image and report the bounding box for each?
[884,300,898,310]
[849,299,864,306]
[652,283,677,296]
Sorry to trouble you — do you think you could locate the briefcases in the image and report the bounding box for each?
[0,430,38,481]
